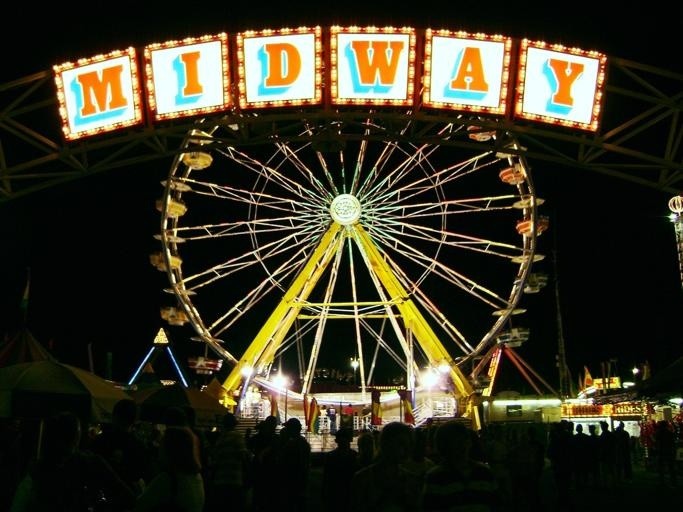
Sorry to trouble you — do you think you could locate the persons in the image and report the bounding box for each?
[1,395,682,510]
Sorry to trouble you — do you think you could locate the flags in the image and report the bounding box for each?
[371,388,383,426]
[397,389,415,427]
